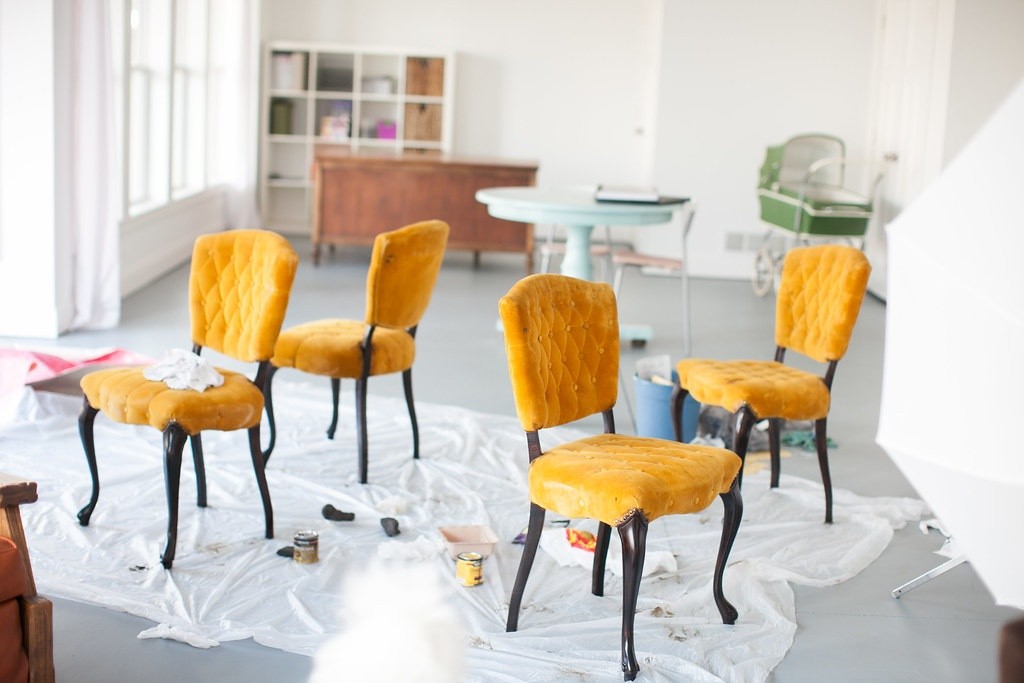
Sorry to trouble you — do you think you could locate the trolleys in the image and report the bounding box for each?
[749,133,898,301]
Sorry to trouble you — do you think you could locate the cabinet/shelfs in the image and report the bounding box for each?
[262,41,458,237]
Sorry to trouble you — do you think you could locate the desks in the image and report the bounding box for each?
[474,184,687,348]
[311,147,539,278]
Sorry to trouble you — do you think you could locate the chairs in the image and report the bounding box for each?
[540,226,615,284]
[613,207,696,354]
[75,228,299,570]
[668,244,873,525]
[260,218,451,484]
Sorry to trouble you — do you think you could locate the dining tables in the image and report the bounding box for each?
[498,272,743,683]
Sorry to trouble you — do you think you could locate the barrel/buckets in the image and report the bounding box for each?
[634,369,700,444]
